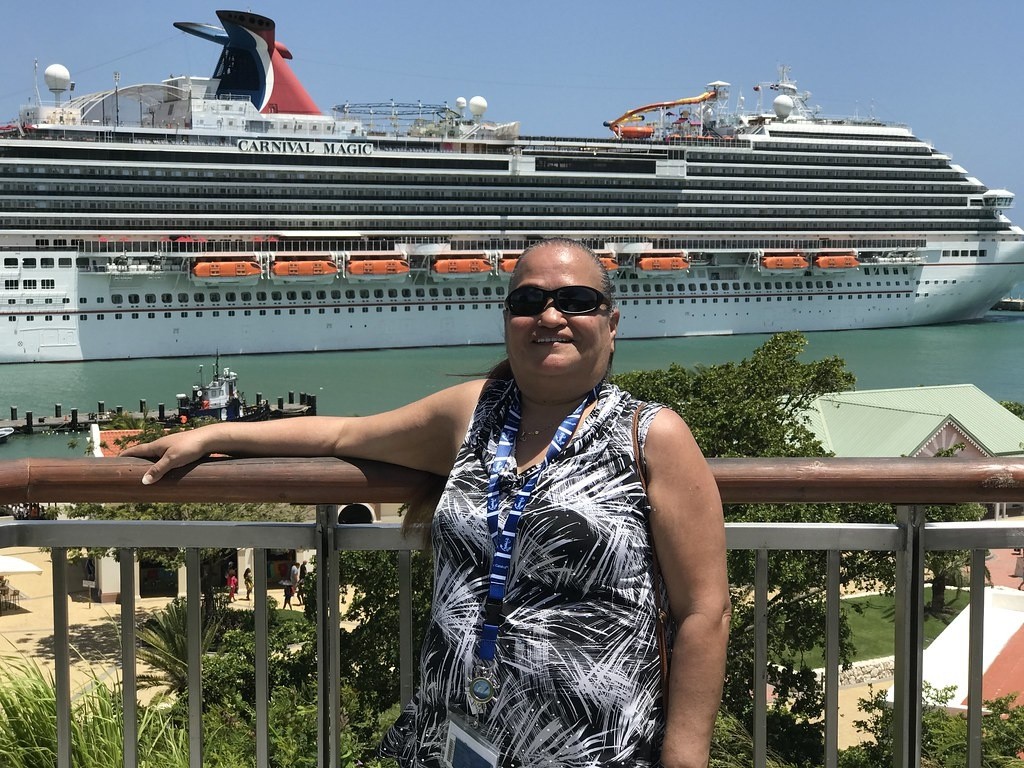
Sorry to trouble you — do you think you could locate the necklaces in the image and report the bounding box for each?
[515,403,579,442]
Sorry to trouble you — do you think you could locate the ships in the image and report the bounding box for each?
[3,9,1023,371]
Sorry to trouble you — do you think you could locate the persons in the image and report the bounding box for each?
[296,574,306,606]
[13,503,45,520]
[117,238,734,768]
[298,559,308,588]
[226,569,238,603]
[282,584,294,611]
[291,562,300,593]
[243,568,254,600]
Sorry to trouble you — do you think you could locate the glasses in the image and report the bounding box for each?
[502,284,612,316]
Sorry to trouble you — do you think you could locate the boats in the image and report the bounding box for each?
[148,350,271,430]
